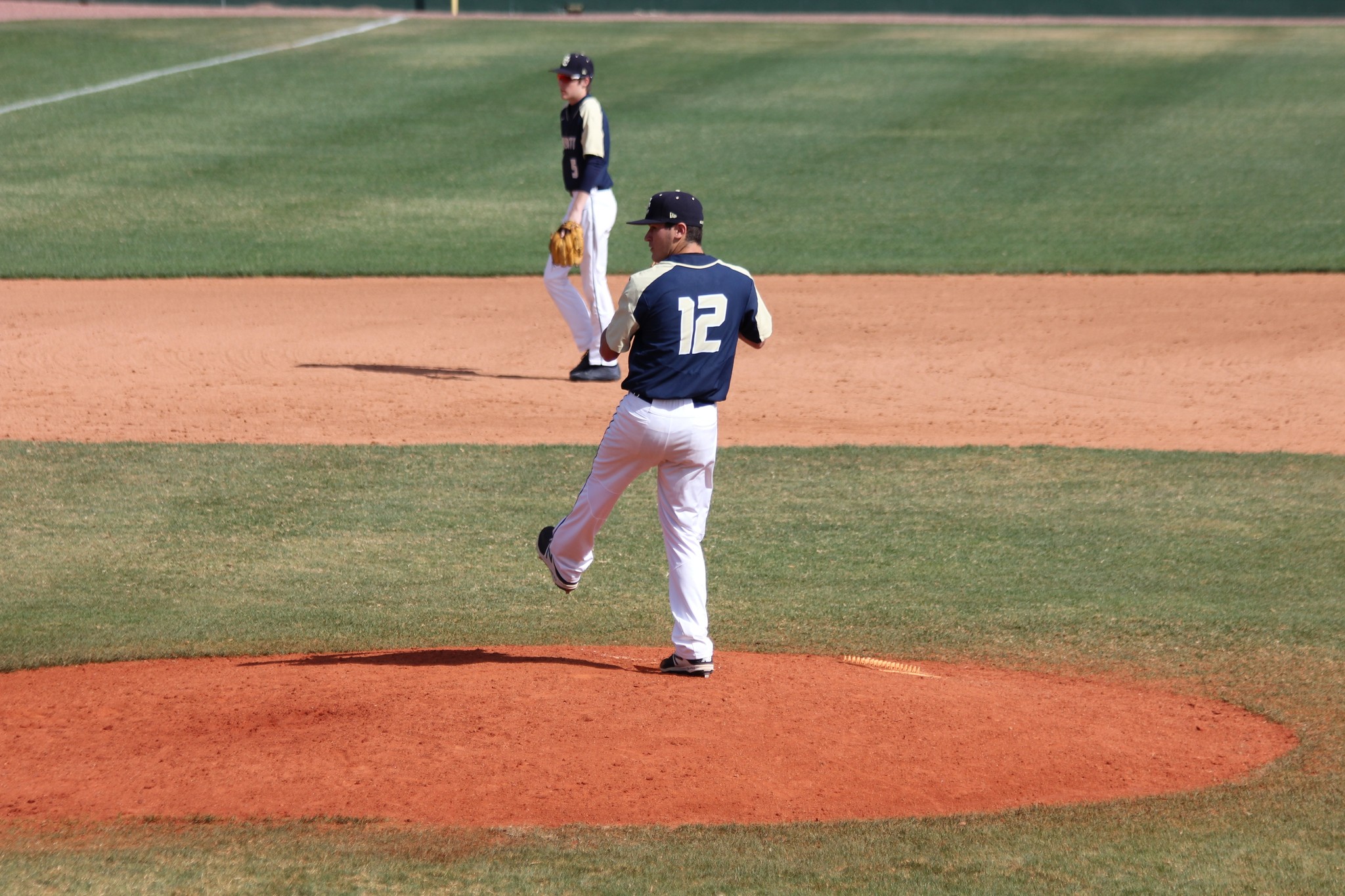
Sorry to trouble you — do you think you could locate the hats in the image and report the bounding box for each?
[626,190,703,228]
[548,53,594,81]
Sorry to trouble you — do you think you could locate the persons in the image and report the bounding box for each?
[537,191,775,677]
[543,53,620,382]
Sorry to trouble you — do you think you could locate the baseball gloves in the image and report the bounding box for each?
[549,221,584,268]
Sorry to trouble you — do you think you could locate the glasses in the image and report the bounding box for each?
[557,74,586,83]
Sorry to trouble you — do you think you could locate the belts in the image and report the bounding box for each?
[629,390,714,409]
[598,185,609,191]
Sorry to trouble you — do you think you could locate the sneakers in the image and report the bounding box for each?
[660,653,713,675]
[571,363,621,381]
[570,350,597,376]
[536,526,579,592]
